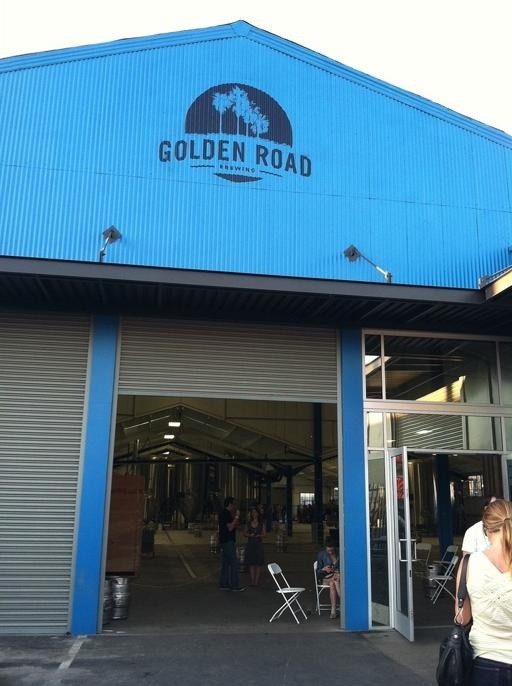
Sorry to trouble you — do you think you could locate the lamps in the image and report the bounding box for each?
[97,223,120,263]
[342,242,392,284]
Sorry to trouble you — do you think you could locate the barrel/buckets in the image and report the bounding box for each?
[424,565,444,598]
[106,575,130,619]
[102,581,113,625]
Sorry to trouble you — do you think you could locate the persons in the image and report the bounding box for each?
[242,507,268,588]
[218,497,247,592]
[433,499,511,685]
[462,496,503,560]
[314,541,340,620]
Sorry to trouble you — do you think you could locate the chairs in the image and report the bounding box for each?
[412,542,459,606]
[267,560,340,623]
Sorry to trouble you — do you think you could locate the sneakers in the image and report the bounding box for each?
[218,583,250,593]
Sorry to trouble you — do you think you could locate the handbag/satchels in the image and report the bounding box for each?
[435,621,474,686]
